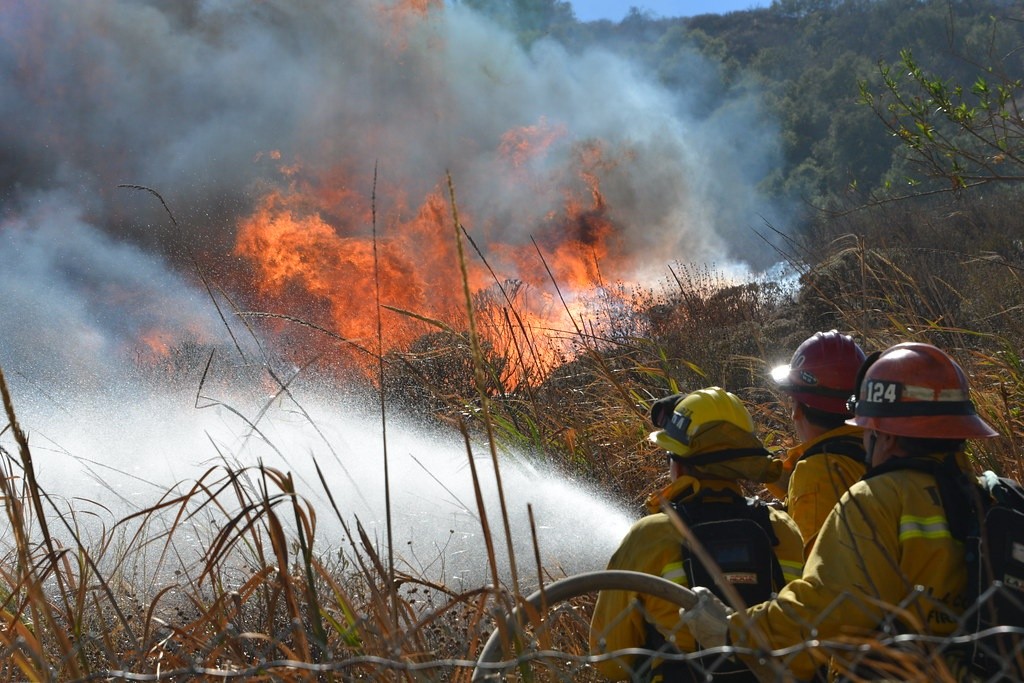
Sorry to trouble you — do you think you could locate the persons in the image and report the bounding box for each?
[590,387,807,683]
[680,329,1024,683]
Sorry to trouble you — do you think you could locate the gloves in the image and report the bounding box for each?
[676,585,734,651]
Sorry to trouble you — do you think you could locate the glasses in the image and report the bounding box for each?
[660,451,679,465]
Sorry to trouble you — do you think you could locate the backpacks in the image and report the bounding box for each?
[936,455,1023,683]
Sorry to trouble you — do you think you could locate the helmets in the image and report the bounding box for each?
[771,328,870,428]
[641,386,786,485]
[844,341,1002,441]
